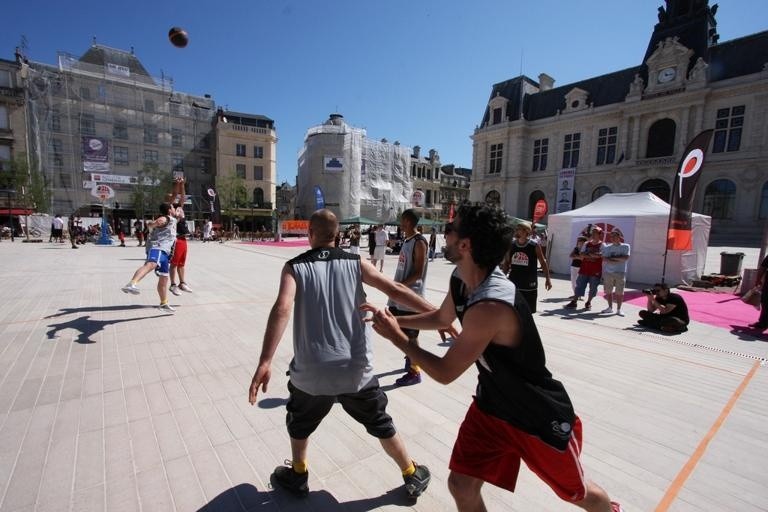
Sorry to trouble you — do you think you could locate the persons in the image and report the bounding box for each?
[429,227,436,262]
[52,176,240,311]
[244,208,461,503]
[599,227,630,317]
[499,223,553,316]
[567,223,604,310]
[358,203,619,512]
[342,223,389,274]
[569,236,585,301]
[640,282,690,334]
[749,254,768,330]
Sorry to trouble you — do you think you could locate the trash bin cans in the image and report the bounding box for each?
[719,251,745,275]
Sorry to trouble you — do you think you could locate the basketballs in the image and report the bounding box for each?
[169,27,189,48]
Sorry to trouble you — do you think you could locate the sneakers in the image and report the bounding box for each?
[403,465,430,499]
[396,371,420,386]
[169,283,192,296]
[566,301,625,316]
[159,304,176,313]
[122,283,140,295]
[275,466,308,497]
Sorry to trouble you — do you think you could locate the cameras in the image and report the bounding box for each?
[642,288,655,294]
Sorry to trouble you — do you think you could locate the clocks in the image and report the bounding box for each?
[656,66,677,84]
